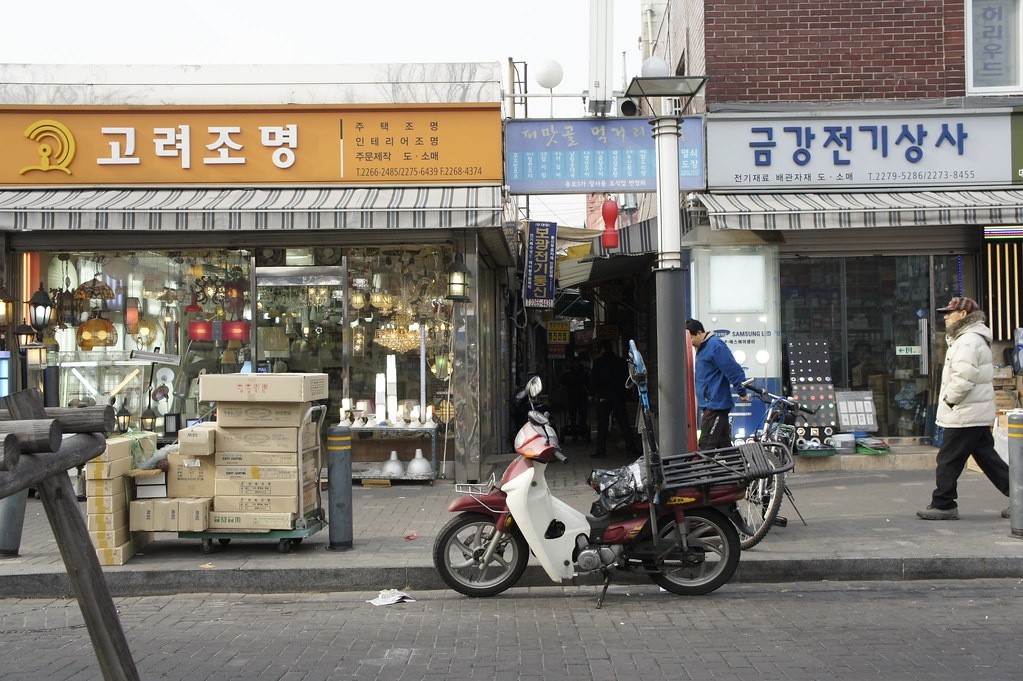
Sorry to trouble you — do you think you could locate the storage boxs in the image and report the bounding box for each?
[932,424,944,447]
[897,409,917,437]
[868,375,892,436]
[86,432,157,566]
[128,373,329,533]
[993,365,1023,430]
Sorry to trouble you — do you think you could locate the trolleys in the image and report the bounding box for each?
[178,402,331,552]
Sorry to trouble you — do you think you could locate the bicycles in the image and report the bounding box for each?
[732,376,824,549]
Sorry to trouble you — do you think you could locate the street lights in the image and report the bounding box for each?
[624,55,708,459]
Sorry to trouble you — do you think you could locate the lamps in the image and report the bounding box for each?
[445,251,471,301]
[0,277,19,339]
[45,245,454,431]
[791,377,836,429]
[13,319,37,357]
[22,282,52,341]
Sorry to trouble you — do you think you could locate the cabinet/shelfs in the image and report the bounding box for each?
[780,256,972,376]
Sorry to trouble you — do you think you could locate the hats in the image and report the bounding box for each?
[936,297,980,314]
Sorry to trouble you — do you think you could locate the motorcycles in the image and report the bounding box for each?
[431,373,793,616]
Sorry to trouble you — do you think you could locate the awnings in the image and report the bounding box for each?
[578,217,657,289]
[698,190,1023,229]
[0,188,505,229]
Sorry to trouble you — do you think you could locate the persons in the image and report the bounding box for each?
[917,297,1009,518]
[513,373,561,448]
[850,339,886,390]
[685,317,749,464]
[590,339,641,457]
[559,358,590,441]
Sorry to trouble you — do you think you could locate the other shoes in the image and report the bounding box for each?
[1001,504,1011,519]
[917,505,959,520]
